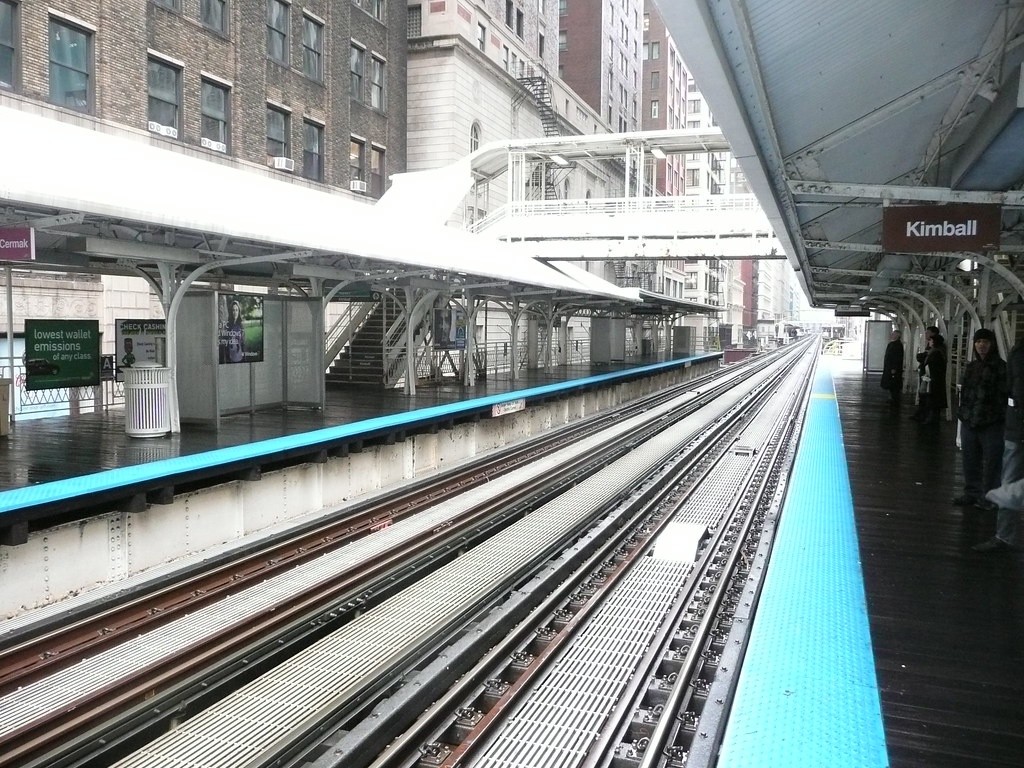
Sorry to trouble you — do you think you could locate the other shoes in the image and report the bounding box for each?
[970,536,1011,554]
[923,417,942,424]
[909,411,926,420]
[975,501,999,511]
[954,491,977,505]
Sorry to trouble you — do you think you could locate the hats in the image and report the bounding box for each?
[930,334,944,344]
[973,329,992,343]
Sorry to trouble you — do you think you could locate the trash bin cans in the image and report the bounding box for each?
[0,378,12,436]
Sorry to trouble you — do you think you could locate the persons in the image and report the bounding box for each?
[223,299,246,363]
[910,326,939,421]
[952,328,1010,512]
[435,309,454,347]
[970,324,1024,554]
[879,330,905,402]
[917,335,947,428]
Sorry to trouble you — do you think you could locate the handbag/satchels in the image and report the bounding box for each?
[883,368,900,384]
[919,365,932,393]
